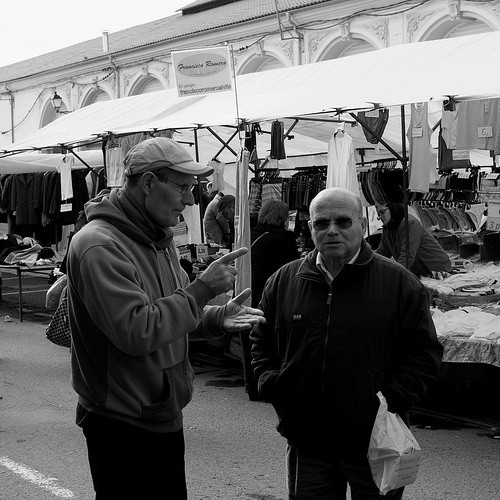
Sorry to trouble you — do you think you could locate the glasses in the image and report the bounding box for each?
[160,177,200,195]
[377,206,391,220]
[308,217,359,230]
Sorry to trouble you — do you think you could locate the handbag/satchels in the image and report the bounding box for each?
[366,392,421,495]
[46,298,75,346]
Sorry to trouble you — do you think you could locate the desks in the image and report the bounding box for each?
[409,335,499,430]
[0,265,60,322]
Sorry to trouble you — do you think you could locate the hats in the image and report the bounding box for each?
[216,194,235,219]
[123,137,214,177]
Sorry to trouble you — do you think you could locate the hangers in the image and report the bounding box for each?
[250,165,328,184]
[407,188,489,209]
[359,157,404,174]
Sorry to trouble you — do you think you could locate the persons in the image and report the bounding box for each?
[64,137,263,500]
[201,187,451,400]
[249,188,443,500]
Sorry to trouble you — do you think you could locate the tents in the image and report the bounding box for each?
[0,32,500,270]
